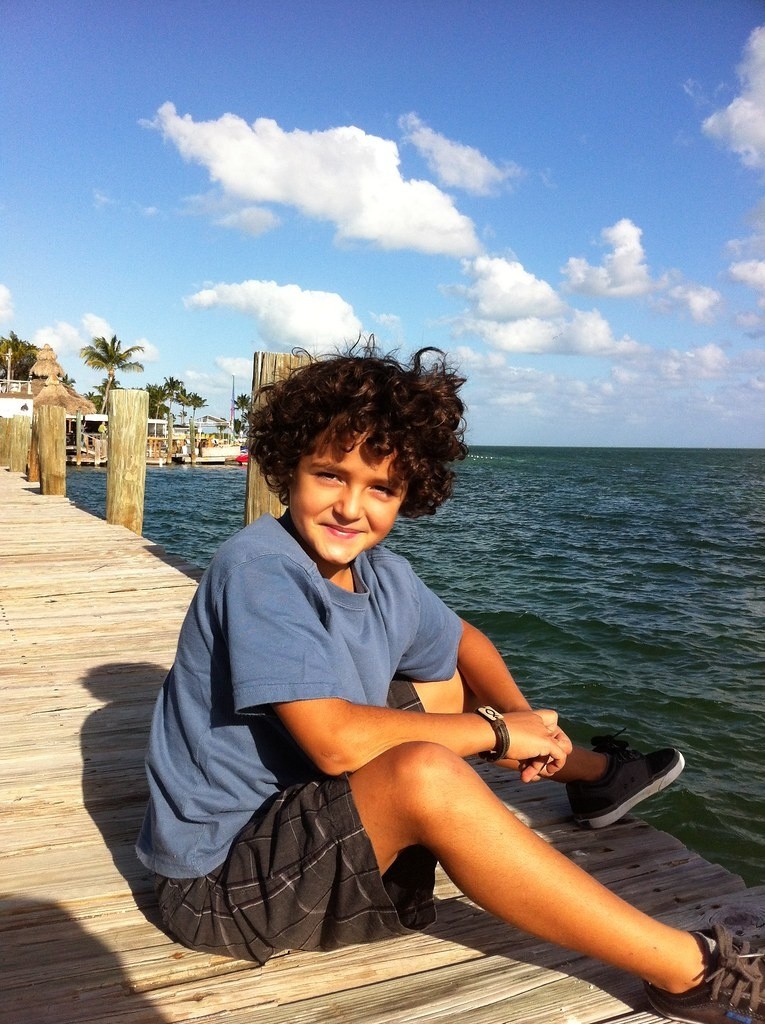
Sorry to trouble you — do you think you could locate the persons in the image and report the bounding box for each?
[133,352,765,1024]
[97,422,107,432]
[81,420,86,445]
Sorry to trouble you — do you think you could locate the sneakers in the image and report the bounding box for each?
[643,924,765,1024]
[566,728,685,829]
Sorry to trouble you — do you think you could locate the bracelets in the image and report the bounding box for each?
[473,703,510,762]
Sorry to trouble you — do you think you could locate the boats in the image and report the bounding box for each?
[225,449,249,466]
